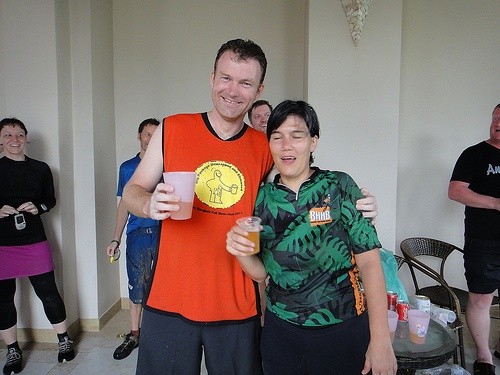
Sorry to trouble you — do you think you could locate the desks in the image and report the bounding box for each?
[385,315,458,375]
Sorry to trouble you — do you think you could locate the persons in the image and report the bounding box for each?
[248,99,274,133]
[106,119,161,360]
[226,99,397,375]
[0,118,76,375]
[122,39,378,375]
[448,104,500,375]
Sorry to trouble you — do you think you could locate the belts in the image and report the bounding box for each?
[133,227,160,234]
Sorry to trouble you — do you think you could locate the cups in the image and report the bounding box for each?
[236,217,261,255]
[387,310,399,344]
[163,171,196,221]
[408,309,430,344]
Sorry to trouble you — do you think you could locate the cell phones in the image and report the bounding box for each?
[14,214,26,230]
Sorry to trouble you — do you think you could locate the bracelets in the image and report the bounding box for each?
[111,240,120,246]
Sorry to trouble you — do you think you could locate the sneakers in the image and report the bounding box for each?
[3,347,22,375]
[113,333,139,360]
[58,336,75,364]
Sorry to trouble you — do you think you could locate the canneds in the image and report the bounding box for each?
[386,291,398,311]
[415,294,430,315]
[396,300,410,322]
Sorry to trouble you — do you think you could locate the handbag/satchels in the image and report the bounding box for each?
[379,247,408,305]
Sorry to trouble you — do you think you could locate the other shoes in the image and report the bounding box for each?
[494,351,500,359]
[473,360,495,375]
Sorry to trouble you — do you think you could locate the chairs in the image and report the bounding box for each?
[394,237,500,368]
